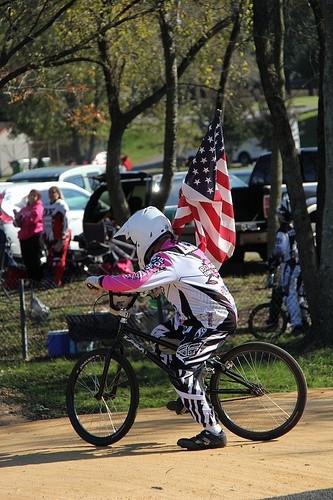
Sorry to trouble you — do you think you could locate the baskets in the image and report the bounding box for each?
[66,312,119,343]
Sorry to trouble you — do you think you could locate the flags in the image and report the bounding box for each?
[173,116,237,275]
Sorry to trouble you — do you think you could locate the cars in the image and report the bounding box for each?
[231,136,270,166]
[0,164,255,273]
[186,146,200,165]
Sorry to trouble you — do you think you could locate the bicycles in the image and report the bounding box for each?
[247,285,311,340]
[64,281,309,446]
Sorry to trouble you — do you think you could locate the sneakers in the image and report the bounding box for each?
[166,398,203,411]
[178,428,227,450]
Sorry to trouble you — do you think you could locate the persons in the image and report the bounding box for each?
[0,186,72,290]
[86,206,237,451]
[263,202,308,337]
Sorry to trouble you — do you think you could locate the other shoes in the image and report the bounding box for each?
[284,325,303,336]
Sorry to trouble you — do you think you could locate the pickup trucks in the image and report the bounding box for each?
[180,146,324,274]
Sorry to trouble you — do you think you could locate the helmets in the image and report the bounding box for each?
[275,198,293,224]
[113,206,175,270]
